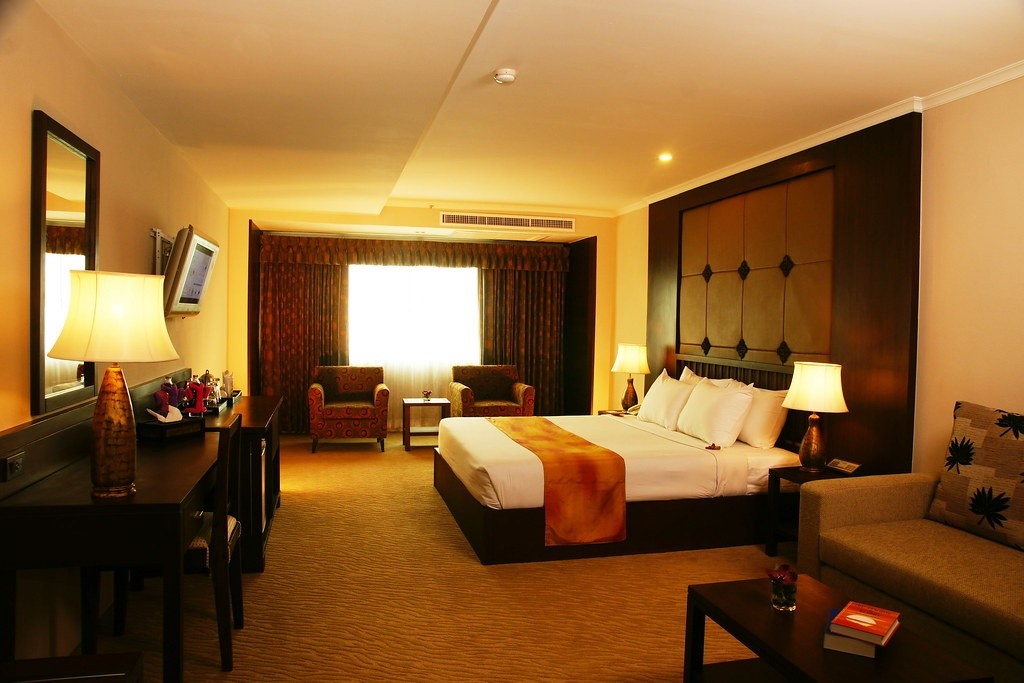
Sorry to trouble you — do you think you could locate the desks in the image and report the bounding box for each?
[402,398,451,452]
[0,432,218,683]
[204,395,284,574]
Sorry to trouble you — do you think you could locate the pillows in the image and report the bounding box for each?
[926,399,1024,552]
[676,376,755,448]
[725,380,788,449]
[637,368,695,432]
[678,365,732,388]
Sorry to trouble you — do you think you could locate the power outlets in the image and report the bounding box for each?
[5,451,26,482]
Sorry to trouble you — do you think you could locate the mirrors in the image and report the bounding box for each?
[29,109,101,417]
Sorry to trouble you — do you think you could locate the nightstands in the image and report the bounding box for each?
[764,467,851,558]
[597,410,628,418]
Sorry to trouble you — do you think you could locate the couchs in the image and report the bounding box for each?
[797,465,1024,665]
[307,366,389,453]
[449,365,535,417]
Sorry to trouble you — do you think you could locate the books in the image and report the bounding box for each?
[823,602,878,658]
[831,602,902,646]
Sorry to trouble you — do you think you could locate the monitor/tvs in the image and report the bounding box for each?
[161,224,220,318]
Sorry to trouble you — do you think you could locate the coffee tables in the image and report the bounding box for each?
[684,573,1009,683]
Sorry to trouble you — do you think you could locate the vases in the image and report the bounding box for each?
[423,395,431,401]
[771,583,796,611]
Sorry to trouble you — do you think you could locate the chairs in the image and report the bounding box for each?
[80,412,242,672]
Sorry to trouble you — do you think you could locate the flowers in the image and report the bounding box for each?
[421,390,432,398]
[765,563,798,608]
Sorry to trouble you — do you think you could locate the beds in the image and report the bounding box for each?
[434,353,827,565]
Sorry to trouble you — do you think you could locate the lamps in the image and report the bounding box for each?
[781,361,849,473]
[48,270,180,499]
[610,343,651,411]
